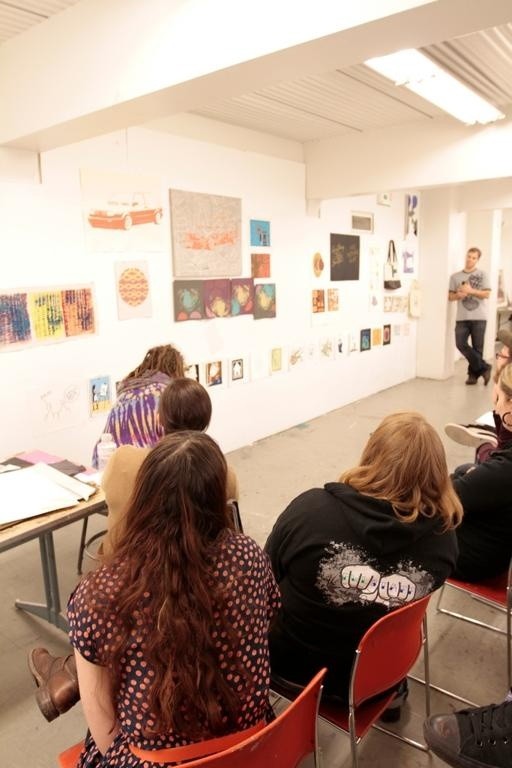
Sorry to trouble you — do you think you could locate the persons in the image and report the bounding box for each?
[97,379,238,564]
[403,217,418,273]
[28,432,281,767]
[444,315,511,449]
[90,345,185,468]
[258,411,464,724]
[385,240,401,289]
[447,363,511,583]
[448,248,491,385]
[422,702,510,768]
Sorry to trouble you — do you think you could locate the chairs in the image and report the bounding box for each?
[269,594,430,768]
[58,667,329,768]
[406,559,512,708]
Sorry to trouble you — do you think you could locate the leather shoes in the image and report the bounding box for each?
[28,647,81,723]
[466,364,491,386]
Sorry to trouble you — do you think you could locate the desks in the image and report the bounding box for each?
[0,451,108,637]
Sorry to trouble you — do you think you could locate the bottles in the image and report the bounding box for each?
[97,434,116,471]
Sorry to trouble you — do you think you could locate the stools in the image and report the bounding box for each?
[78,507,108,575]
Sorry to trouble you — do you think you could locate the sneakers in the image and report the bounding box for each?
[422,688,512,767]
[444,423,500,450]
